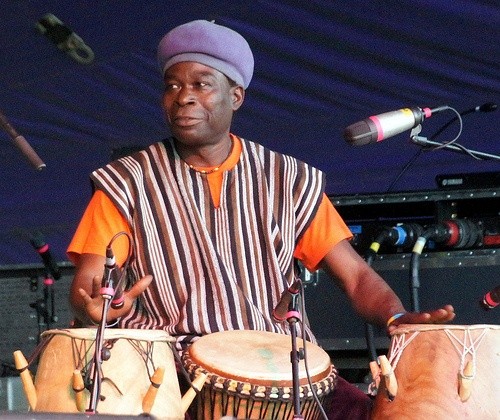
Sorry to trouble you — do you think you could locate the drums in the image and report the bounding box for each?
[11,325,206,419]
[365,322,500,420]
[184,329,339,419]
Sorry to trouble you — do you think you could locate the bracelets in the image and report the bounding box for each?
[386,312,405,327]
[90,316,122,328]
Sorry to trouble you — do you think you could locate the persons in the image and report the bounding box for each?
[65,19,456,420]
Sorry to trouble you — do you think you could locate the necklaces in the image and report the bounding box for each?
[184,135,234,174]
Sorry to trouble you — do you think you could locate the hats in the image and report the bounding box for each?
[156,20,255,90]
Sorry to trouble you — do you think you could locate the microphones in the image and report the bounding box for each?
[386,103,498,192]
[30,238,62,280]
[106,246,125,309]
[272,282,301,323]
[344,105,449,146]
